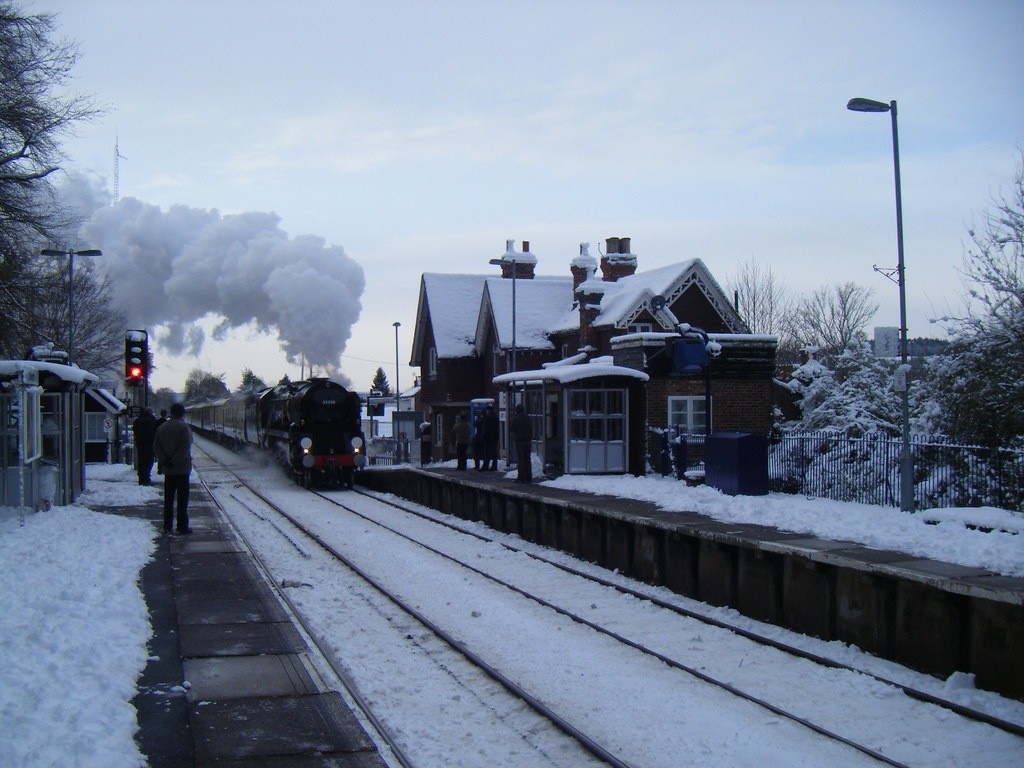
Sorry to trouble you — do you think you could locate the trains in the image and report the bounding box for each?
[184,377,366,490]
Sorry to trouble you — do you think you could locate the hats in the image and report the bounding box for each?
[514,404,524,411]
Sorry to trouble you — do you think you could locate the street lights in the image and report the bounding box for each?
[651,295,710,436]
[846,98,916,514]
[41,249,103,367]
[489,259,516,416]
[392,322,401,465]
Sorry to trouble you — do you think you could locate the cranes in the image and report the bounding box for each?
[113,135,128,203]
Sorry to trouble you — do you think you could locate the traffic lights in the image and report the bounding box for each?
[125,329,147,387]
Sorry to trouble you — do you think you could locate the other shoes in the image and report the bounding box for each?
[177,527,193,534]
[165,528,173,534]
[511,479,527,483]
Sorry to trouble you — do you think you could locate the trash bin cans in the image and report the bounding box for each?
[704,432,770,496]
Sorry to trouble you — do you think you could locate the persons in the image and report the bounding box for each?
[157,410,167,474]
[134,407,155,485]
[510,404,532,482]
[453,415,470,471]
[471,407,499,470]
[152,404,194,536]
